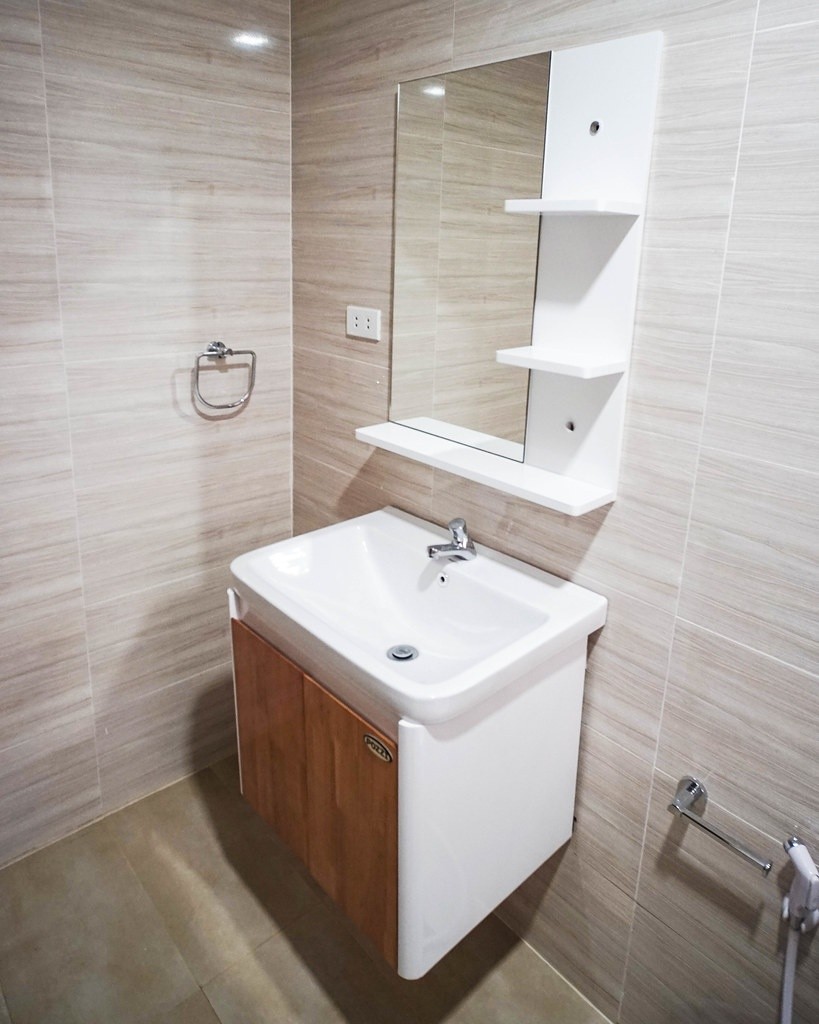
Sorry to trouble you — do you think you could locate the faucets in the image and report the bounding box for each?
[426,517,478,562]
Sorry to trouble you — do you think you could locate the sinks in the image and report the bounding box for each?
[229,504,609,725]
